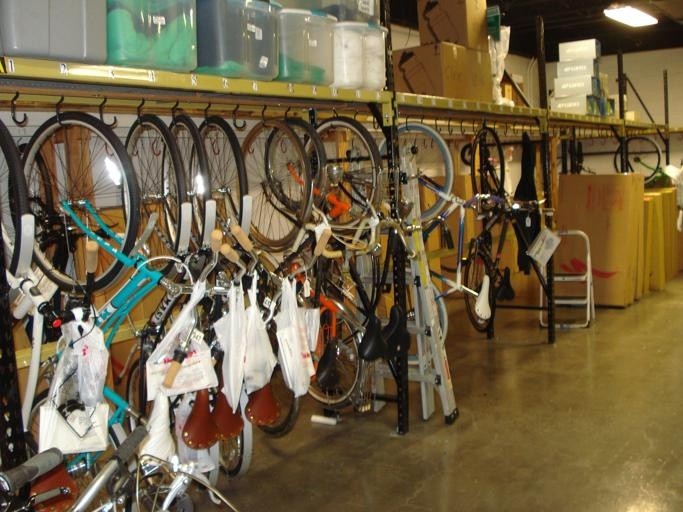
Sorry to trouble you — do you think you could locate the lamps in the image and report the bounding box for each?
[603,6,658,28]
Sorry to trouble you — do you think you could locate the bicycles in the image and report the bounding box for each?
[9,111,209,511]
[266,118,359,405]
[0,426,236,512]
[240,119,342,400]
[163,115,281,476]
[120,116,220,496]
[0,121,71,512]
[378,123,506,366]
[25,349,146,476]
[188,117,301,439]
[464,131,554,331]
[557,137,662,185]
[304,117,420,406]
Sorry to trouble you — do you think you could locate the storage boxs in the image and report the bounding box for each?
[601,97,610,116]
[511,74,525,106]
[559,38,601,61]
[599,72,609,99]
[335,21,388,91]
[551,96,601,117]
[554,76,601,99]
[616,111,640,122]
[196,1,283,81]
[279,8,337,87]
[393,42,469,99]
[608,94,627,112]
[107,1,197,74]
[557,59,599,79]
[501,81,512,100]
[418,0,489,52]
[467,49,493,102]
[1,0,108,65]
